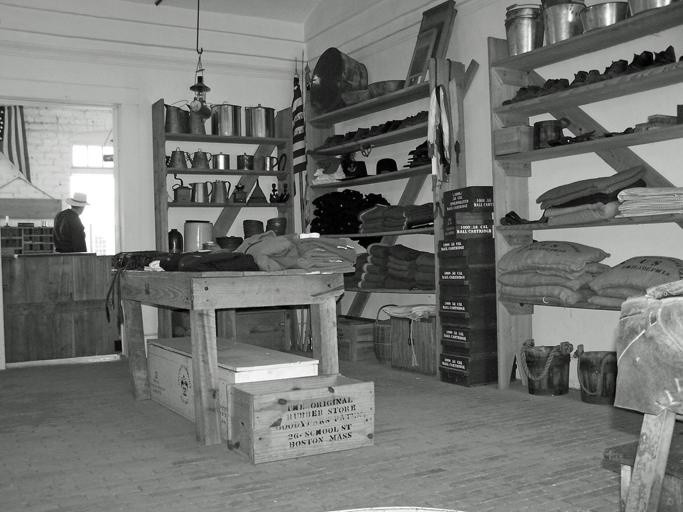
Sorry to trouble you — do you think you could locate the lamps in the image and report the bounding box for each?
[189,0,210,103]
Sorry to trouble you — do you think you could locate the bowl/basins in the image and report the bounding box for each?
[578,0,672,33]
[216,236,244,251]
[310,47,405,113]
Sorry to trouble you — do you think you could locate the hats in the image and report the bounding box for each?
[377,159,397,175]
[336,161,375,181]
[66,193,90,206]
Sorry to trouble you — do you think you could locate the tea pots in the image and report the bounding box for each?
[257,153,278,173]
[189,179,232,204]
[185,148,213,170]
[164,146,188,171]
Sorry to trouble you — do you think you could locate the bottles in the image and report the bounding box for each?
[203,240,216,251]
[168,229,183,253]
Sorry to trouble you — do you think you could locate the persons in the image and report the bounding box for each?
[54,192,90,253]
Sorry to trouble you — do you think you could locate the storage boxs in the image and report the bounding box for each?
[145,333,374,465]
[337,313,436,374]
[437,185,496,389]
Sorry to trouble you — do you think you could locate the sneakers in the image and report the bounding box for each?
[316,111,427,150]
[502,46,675,105]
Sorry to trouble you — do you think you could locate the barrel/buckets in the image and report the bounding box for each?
[544,4,587,45]
[504,3,545,56]
[521,337,573,398]
[576,343,617,402]
[182,222,214,252]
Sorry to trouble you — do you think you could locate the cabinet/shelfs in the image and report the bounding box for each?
[151,97,295,252]
[303,60,465,378]
[487,0,679,407]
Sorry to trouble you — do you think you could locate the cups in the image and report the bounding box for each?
[212,152,230,170]
[237,153,254,170]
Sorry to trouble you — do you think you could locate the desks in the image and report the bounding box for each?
[111,267,355,447]
